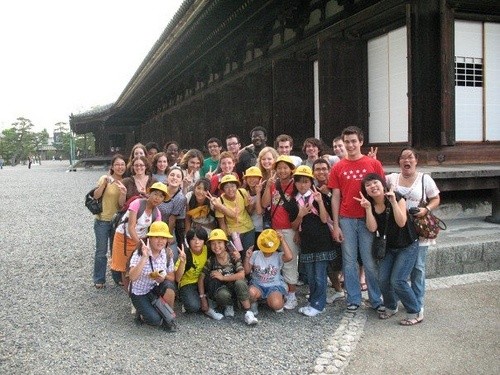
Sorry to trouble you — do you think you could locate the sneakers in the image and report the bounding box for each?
[182,276,425,325]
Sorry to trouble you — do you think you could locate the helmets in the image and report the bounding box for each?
[257,229,280,253]
[149,182,169,196]
[242,167,263,181]
[272,155,295,170]
[294,165,315,179]
[146,220,173,238]
[207,229,229,243]
[219,175,241,190]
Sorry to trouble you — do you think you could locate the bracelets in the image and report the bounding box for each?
[426,206,430,211]
[200,295,206,298]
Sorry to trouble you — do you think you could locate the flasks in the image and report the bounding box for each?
[232,232,243,251]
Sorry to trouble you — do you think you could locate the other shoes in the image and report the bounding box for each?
[118,280,125,286]
[96,283,106,288]
[137,316,145,324]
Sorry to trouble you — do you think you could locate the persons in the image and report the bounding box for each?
[322,136,348,168]
[301,137,323,188]
[368,146,439,316]
[312,159,345,304]
[353,172,423,325]
[326,126,386,314]
[93,154,127,288]
[111,127,306,326]
[76,148,80,160]
[28,157,32,169]
[288,164,333,317]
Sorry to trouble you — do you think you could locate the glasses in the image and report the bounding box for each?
[113,164,126,167]
[209,145,220,148]
[133,164,145,167]
[227,142,239,146]
[314,168,328,171]
[250,135,262,138]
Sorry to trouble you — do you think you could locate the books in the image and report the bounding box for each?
[148,270,164,279]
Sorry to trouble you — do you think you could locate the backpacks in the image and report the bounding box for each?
[111,194,157,231]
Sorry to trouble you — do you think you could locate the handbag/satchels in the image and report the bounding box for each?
[86,187,102,214]
[413,173,447,239]
[151,296,177,327]
[373,237,387,260]
[284,199,298,222]
